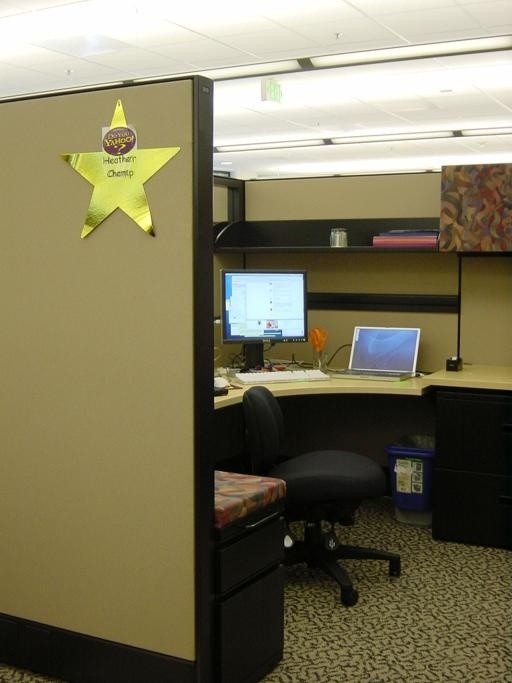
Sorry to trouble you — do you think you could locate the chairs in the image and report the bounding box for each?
[240,385,400,605]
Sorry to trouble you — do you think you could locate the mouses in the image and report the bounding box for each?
[214,377,230,388]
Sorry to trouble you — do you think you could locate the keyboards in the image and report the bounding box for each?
[235,369,330,385]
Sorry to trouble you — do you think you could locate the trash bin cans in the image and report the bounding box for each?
[384,432,435,511]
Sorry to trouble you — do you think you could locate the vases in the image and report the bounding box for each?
[313,350,328,374]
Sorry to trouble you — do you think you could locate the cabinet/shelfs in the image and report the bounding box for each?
[432,387,512,550]
[214,470,287,683]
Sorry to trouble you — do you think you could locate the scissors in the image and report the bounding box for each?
[309,328,327,369]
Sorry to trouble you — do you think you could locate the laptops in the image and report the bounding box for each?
[331,326,421,382]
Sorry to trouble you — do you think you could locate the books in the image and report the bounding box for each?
[371,224,440,249]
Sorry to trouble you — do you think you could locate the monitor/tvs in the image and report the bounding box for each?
[219,268,308,373]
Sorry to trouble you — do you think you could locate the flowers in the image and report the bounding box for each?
[309,328,328,358]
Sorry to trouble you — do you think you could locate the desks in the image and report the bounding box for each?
[212,371,433,410]
